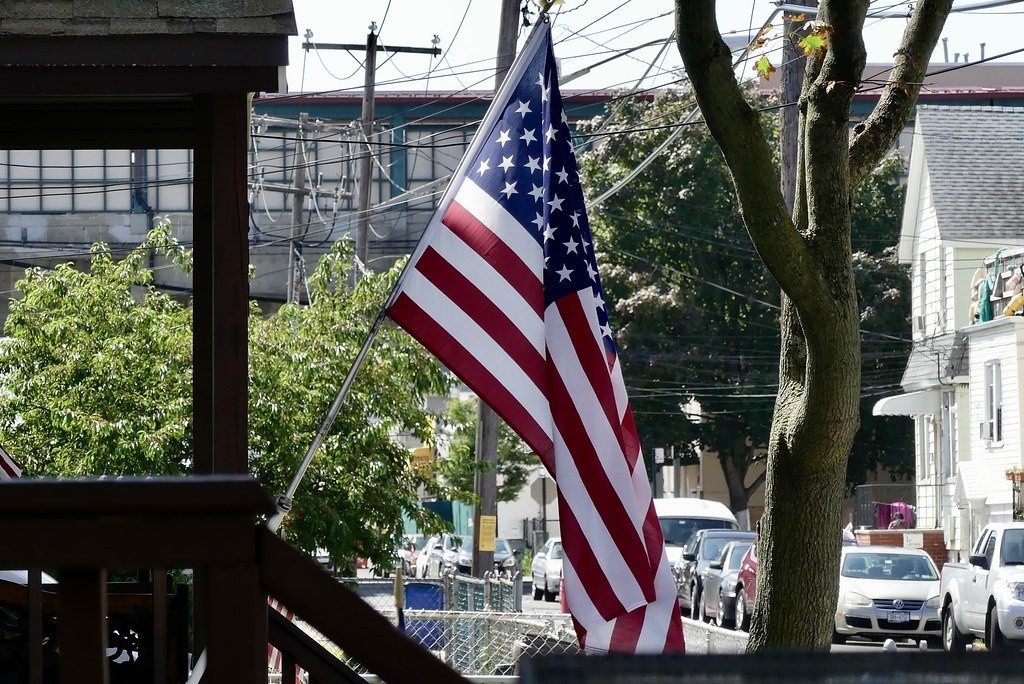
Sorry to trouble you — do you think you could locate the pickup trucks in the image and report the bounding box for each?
[937,521,1024,653]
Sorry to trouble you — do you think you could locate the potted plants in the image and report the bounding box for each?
[1014,465,1024,481]
[1004,465,1018,480]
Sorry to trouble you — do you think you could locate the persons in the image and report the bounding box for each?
[887,512,908,530]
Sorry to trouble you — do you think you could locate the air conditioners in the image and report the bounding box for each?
[914,314,924,333]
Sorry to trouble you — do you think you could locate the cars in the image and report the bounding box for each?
[382,533,465,580]
[451,535,521,580]
[698,540,752,628]
[530,538,562,601]
[734,540,760,631]
[671,527,757,621]
[832,546,942,648]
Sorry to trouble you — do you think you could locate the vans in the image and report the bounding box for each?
[652,498,743,571]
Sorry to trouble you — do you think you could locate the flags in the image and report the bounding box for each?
[387,5,691,657]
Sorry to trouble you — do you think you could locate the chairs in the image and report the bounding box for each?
[868,567,885,577]
[1005,542,1020,561]
[847,558,868,577]
[897,560,914,579]
